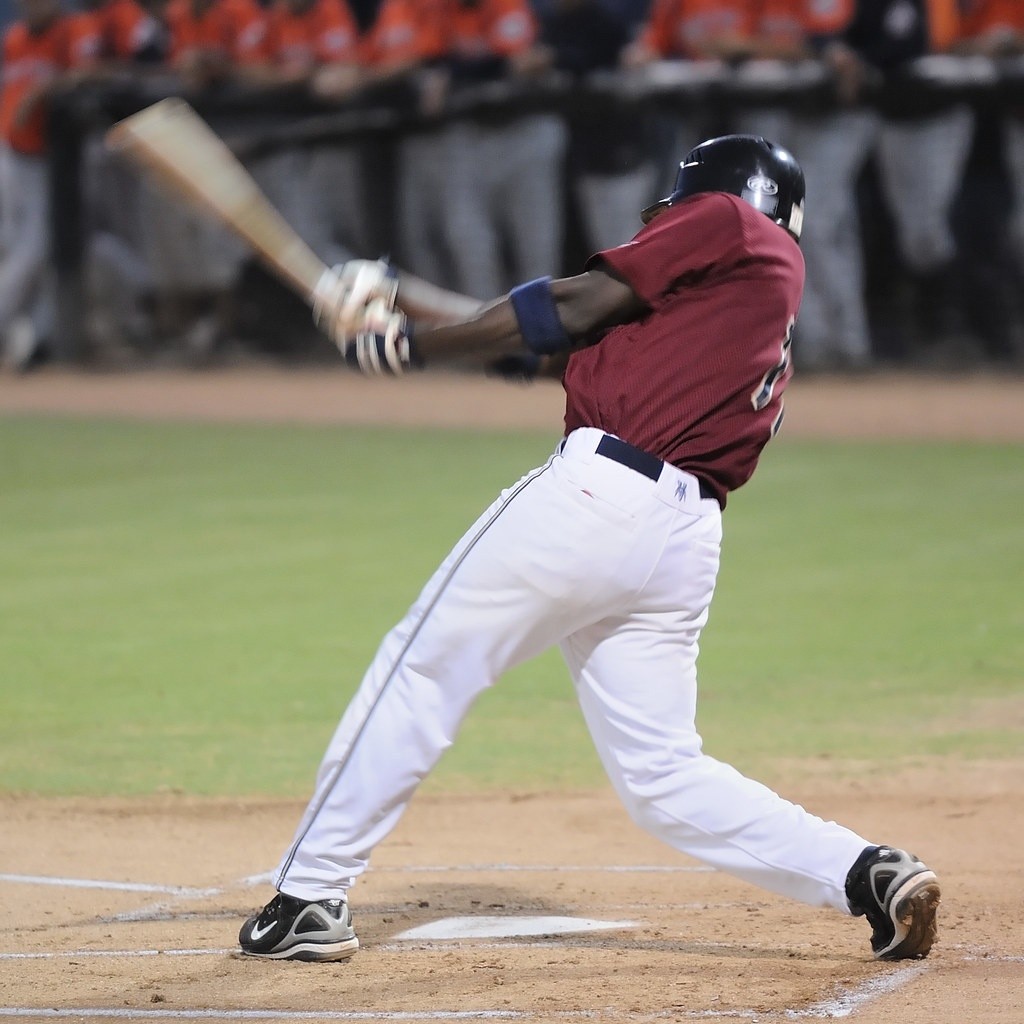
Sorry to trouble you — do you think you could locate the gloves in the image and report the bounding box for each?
[310,251,424,376]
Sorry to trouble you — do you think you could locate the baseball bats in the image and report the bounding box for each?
[97,94,413,374]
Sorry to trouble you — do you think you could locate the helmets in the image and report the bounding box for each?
[640,134,806,246]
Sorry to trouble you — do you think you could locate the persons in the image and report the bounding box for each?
[0,0,1024,371]
[240,136,938,962]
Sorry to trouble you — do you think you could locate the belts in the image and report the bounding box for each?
[559,434,723,510]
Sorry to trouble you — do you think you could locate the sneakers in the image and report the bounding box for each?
[238,892,360,963]
[859,844,941,960]
